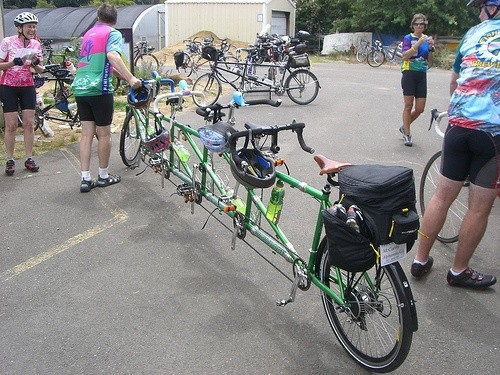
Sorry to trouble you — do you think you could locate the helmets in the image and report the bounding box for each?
[14,12,38,27]
[230,148,276,189]
[466,0,500,8]
[127,85,151,106]
[144,128,169,153]
[200,129,230,152]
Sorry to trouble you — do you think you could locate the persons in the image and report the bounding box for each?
[399,14,434,146]
[70,4,142,191]
[0,11,44,175]
[411,0,500,288]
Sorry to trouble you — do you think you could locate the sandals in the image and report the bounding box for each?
[80,177,97,192]
[96,173,121,187]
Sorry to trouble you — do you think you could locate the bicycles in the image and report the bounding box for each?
[132,39,160,71]
[116,69,422,374]
[366,39,402,68]
[106,67,120,93]
[355,38,386,66]
[37,38,79,82]
[16,63,113,147]
[191,46,322,108]
[175,36,242,83]
[419,107,469,245]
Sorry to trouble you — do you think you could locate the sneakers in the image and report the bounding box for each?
[447,267,497,289]
[5,160,15,174]
[403,133,413,145]
[399,126,404,134]
[411,256,433,276]
[25,158,39,172]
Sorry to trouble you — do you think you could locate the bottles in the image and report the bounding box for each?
[36,92,45,109]
[266,180,285,221]
[66,61,77,76]
[146,124,156,135]
[224,187,246,214]
[173,137,190,161]
[262,79,272,84]
[227,67,243,74]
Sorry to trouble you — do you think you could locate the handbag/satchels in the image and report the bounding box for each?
[290,53,311,68]
[173,52,183,69]
[202,46,217,61]
[322,164,419,272]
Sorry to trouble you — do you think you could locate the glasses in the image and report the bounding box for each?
[413,22,426,26]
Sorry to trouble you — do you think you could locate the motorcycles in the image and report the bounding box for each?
[246,24,311,65]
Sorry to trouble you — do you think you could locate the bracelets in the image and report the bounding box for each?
[411,47,416,52]
